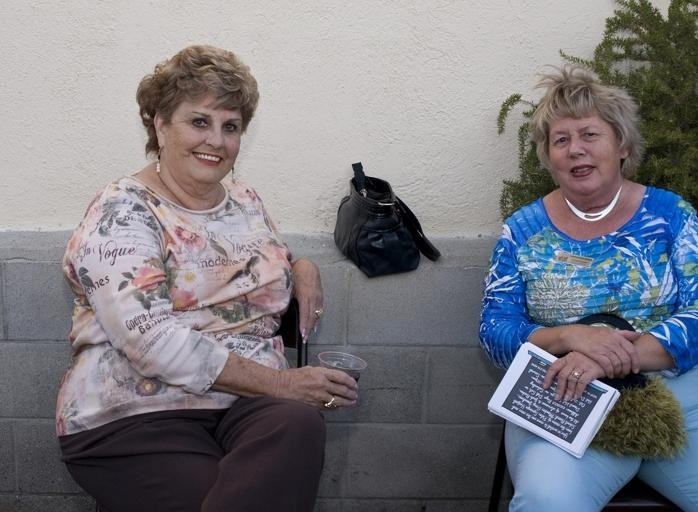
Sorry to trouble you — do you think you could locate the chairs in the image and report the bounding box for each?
[93,291,311,512]
[487,415,674,512]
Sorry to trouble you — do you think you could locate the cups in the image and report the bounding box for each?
[318,350,369,384]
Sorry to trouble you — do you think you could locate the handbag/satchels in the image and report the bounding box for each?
[333,161,442,280]
[553,311,690,461]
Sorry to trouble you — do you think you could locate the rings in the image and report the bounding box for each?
[323,393,335,410]
[314,309,323,317]
[570,371,582,380]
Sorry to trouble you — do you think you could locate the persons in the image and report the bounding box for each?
[52,39,361,512]
[477,61,697,511]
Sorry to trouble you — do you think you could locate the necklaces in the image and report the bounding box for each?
[562,183,623,223]
